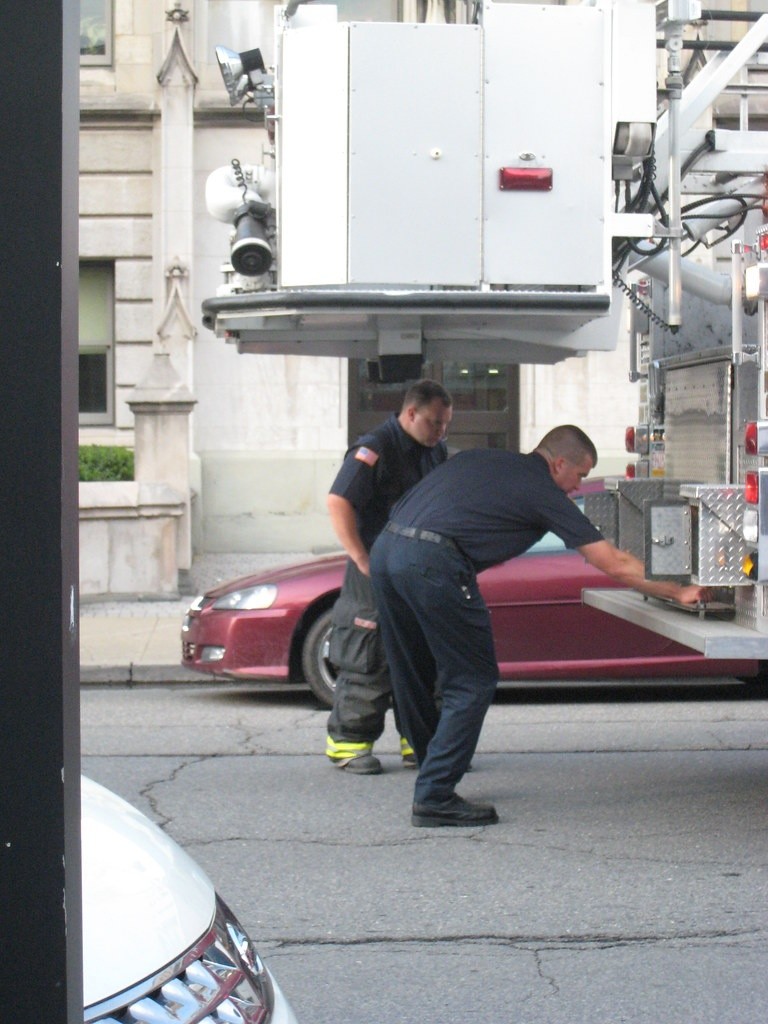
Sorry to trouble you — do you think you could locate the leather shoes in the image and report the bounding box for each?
[412,793,498,827]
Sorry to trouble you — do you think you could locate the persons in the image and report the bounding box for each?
[322,379,454,774]
[369,424,715,827]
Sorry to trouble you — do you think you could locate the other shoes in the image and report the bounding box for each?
[402,754,473,772]
[330,755,383,774]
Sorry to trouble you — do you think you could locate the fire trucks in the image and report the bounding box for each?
[202,1,767,663]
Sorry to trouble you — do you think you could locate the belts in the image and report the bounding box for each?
[386,521,457,551]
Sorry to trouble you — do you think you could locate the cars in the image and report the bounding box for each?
[81,777,298,1024]
[180,474,768,711]
